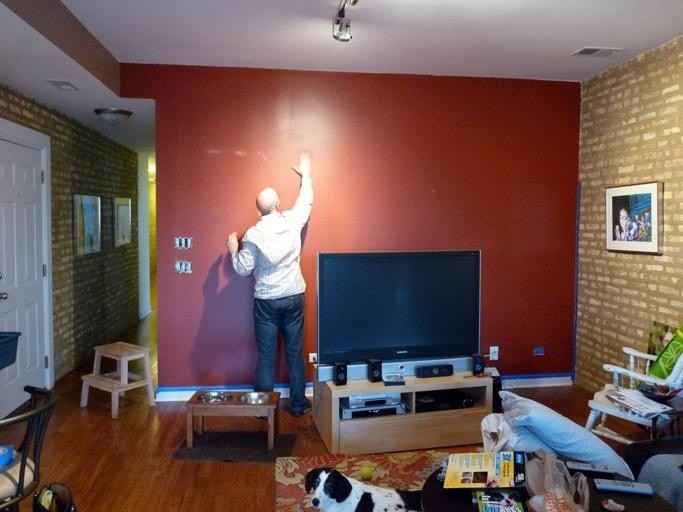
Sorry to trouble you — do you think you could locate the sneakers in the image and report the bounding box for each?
[289,399,312,417]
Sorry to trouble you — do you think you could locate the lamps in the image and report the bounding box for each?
[329,0,360,43]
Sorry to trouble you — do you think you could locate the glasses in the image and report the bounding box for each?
[619,215,628,220]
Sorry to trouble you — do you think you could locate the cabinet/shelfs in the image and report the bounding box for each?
[310,362,492,457]
[78,340,155,421]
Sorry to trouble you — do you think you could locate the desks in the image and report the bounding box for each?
[418,459,676,511]
[183,389,280,450]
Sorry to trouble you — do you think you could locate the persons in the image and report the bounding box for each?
[611,206,652,243]
[224,150,316,420]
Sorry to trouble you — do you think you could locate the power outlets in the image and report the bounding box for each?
[489,346,498,361]
[308,353,317,362]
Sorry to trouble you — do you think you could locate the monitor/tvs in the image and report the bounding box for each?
[317,249,482,364]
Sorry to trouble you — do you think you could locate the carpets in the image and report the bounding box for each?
[272,446,482,512]
[173,430,296,463]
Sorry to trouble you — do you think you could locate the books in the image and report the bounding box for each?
[470,488,525,511]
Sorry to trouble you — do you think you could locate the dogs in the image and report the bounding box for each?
[304,465,423,512]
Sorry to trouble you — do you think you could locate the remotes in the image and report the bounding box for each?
[567,462,615,473]
[594,479,653,494]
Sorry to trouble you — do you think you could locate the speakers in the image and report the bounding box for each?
[416,365,453,377]
[472,353,484,375]
[332,362,347,386]
[368,359,382,383]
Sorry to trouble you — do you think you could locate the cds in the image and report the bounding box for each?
[386,374,400,380]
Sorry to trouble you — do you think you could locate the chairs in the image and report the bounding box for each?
[619,436,682,512]
[584,346,682,445]
[0,384,59,512]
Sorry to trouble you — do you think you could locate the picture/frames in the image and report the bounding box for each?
[111,196,132,247]
[71,191,103,258]
[603,179,664,256]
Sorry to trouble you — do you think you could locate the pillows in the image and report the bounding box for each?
[633,324,682,388]
[496,387,637,480]
[478,410,560,458]
